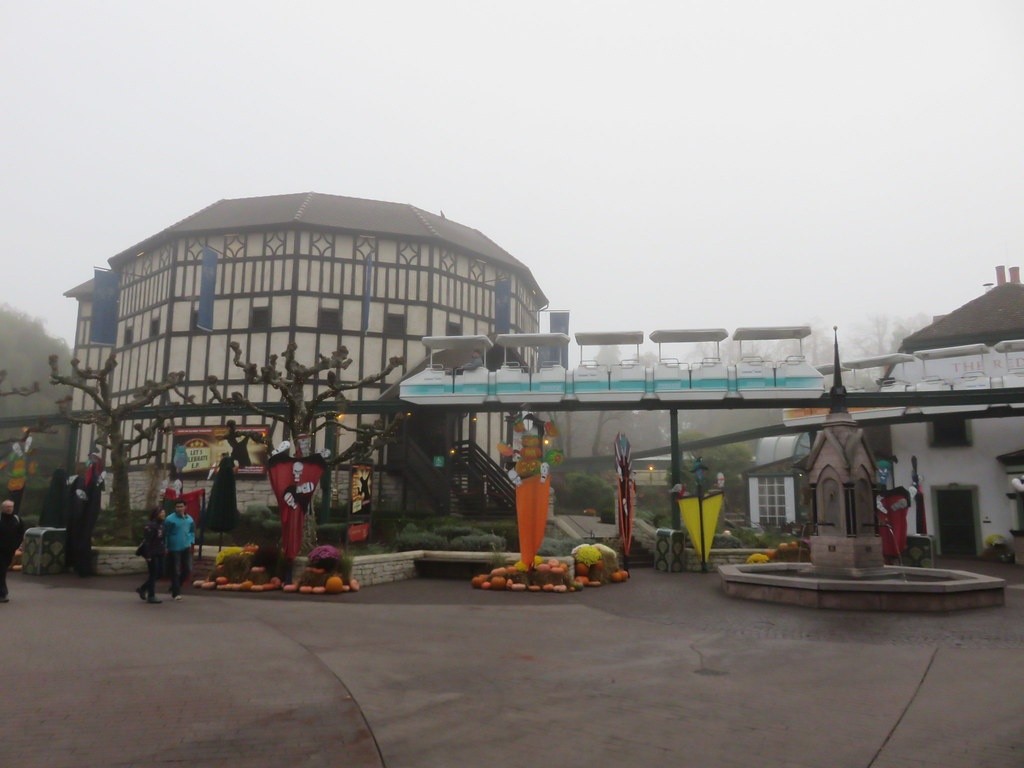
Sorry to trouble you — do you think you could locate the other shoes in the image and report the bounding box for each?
[174,595,184,601]
[136,587,145,600]
[147,598,163,603]
[0,599,9,603]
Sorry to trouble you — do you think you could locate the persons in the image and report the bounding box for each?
[251,525,292,587]
[136,500,195,604]
[0,500,25,603]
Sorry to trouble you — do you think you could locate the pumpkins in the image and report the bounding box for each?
[193,566,359,592]
[472,556,628,592]
